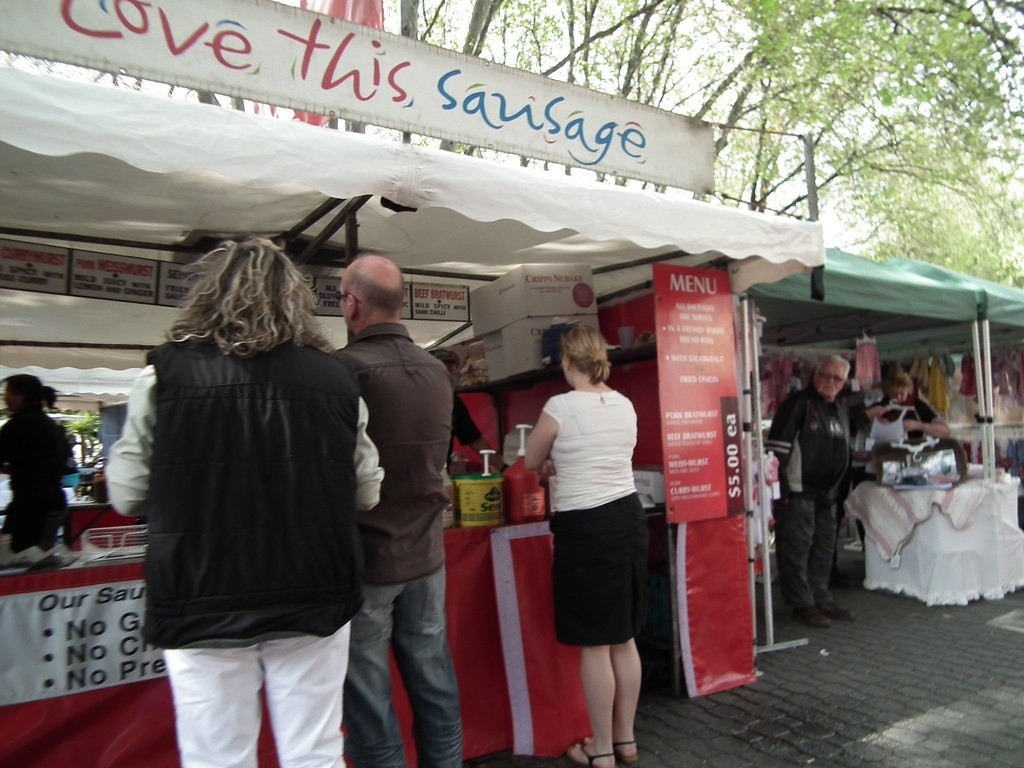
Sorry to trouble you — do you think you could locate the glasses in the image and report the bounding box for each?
[334,290,362,304]
[815,372,845,385]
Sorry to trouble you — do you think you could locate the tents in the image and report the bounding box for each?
[0,58,1024,657]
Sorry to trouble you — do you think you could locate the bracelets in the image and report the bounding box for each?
[500,463,508,474]
[922,422,925,431]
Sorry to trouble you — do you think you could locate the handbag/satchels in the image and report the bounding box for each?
[874,434,968,486]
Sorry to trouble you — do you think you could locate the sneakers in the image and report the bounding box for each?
[792,606,833,629]
[818,604,857,620]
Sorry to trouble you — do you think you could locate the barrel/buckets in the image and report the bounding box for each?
[451,473,505,527]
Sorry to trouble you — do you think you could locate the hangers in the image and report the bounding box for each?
[856,327,878,347]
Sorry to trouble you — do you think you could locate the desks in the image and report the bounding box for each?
[1,507,574,768]
[844,464,1020,606]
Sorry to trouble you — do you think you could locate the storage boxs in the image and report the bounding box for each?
[469,263,601,383]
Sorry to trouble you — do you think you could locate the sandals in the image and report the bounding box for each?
[564,744,618,768]
[612,740,639,763]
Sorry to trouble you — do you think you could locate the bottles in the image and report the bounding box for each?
[502,455,547,523]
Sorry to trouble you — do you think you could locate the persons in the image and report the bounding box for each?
[0,374,80,552]
[427,349,511,477]
[101,236,385,768]
[863,370,951,459]
[524,324,650,768]
[326,254,466,768]
[765,355,858,627]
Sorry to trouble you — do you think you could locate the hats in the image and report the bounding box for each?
[430,349,461,366]
